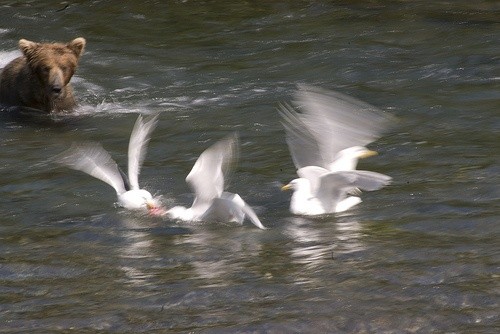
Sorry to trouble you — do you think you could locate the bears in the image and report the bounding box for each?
[0,37,85,114]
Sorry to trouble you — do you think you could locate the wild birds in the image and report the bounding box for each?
[280,165,394,215]
[278,83,399,171]
[51,113,160,212]
[157,138,271,230]
